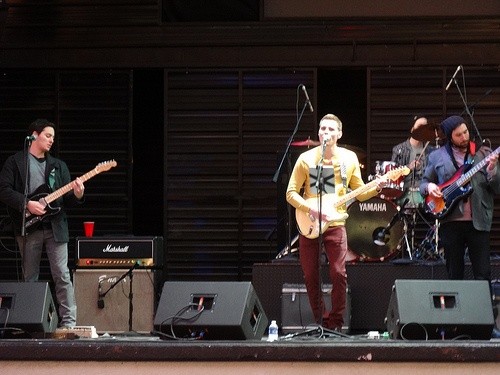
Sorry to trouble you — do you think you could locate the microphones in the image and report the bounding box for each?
[26,135,37,140]
[302,85,314,112]
[378,229,386,238]
[434,129,440,149]
[446,66,460,90]
[98,284,104,309]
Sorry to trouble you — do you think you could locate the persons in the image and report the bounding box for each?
[393,113,442,210]
[419,116,500,339]
[286,114,387,337]
[0,119,86,338]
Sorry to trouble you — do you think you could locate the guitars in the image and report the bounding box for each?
[24,159,118,228]
[295,164,411,239]
[426,146,500,218]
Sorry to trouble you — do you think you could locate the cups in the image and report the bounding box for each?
[84,222,94,238]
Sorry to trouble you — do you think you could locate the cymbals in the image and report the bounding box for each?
[292,140,320,147]
[336,144,367,160]
[411,124,444,141]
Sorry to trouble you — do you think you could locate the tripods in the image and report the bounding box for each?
[279,142,352,340]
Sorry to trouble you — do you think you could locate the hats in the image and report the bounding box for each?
[440,116,465,140]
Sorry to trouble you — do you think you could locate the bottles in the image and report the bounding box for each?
[268,320,278,341]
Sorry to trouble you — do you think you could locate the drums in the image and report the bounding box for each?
[375,161,404,200]
[343,196,408,260]
[396,187,428,211]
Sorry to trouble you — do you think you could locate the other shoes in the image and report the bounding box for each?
[310,328,328,337]
[328,326,342,337]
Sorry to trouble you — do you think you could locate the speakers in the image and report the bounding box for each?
[282,284,351,333]
[0,279,59,339]
[154,281,269,340]
[386,280,494,340]
[73,269,161,337]
